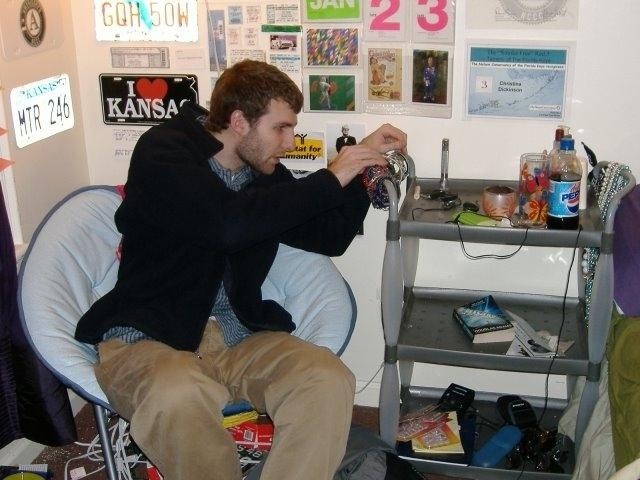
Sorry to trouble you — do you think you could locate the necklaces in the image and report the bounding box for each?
[579,161,630,331]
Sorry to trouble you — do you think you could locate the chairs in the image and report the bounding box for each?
[17,184,358,480]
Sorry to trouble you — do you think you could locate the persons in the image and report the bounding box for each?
[315,75,333,105]
[423,57,438,101]
[336,125,357,152]
[76,59,408,479]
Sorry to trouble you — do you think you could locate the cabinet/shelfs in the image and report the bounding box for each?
[383,172,636,479]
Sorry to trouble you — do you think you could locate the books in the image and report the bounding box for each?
[453,295,514,344]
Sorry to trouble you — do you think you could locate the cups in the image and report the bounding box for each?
[520,153,550,230]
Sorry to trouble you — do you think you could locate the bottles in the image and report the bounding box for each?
[546,137,583,230]
[550,129,565,159]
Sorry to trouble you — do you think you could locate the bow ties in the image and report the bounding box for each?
[342,135,350,138]
